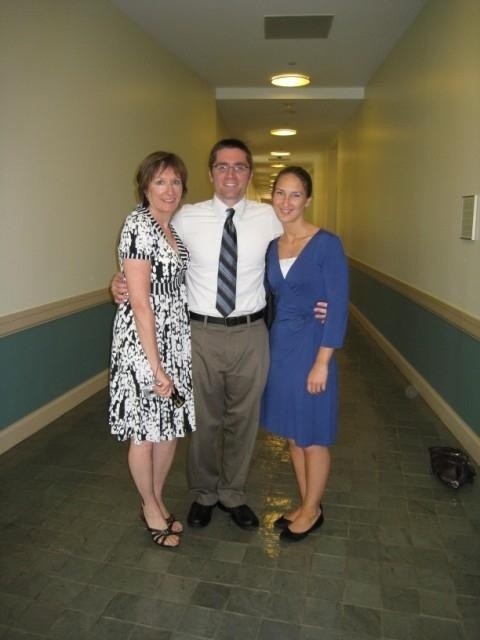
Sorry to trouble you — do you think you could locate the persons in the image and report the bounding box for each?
[107,138,329,531]
[259,164,351,545]
[105,151,200,550]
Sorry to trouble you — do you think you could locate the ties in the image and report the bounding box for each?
[215,207,238,318]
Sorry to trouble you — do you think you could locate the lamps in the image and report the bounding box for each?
[271,72,312,88]
[269,129,297,179]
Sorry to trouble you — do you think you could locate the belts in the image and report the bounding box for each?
[190,310,264,326]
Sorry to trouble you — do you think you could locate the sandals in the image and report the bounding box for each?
[139,504,181,549]
[165,513,184,535]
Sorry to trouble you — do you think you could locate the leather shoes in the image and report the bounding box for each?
[187,501,212,527]
[217,500,259,530]
[279,508,324,542]
[273,501,323,529]
